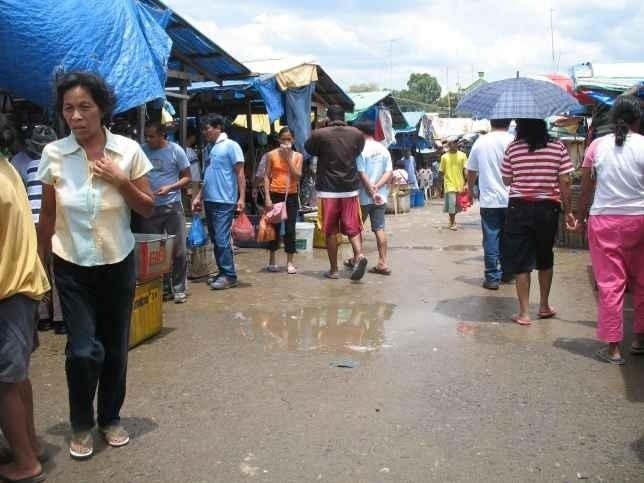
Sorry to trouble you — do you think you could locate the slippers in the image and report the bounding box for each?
[267,264,279,272]
[0,447,48,482]
[101,426,130,446]
[69,432,94,457]
[596,349,625,364]
[537,305,557,317]
[286,262,296,273]
[631,344,644,354]
[512,313,531,324]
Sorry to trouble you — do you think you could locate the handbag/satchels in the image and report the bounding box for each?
[458,192,473,208]
[266,203,288,224]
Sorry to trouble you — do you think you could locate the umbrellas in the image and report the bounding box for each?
[453,70,587,119]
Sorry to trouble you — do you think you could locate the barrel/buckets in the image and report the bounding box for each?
[410,193,416,208]
[295,222,316,254]
[414,191,424,206]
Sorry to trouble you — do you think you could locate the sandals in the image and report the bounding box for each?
[324,255,392,279]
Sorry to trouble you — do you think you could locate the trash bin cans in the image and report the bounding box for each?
[415,192,425,207]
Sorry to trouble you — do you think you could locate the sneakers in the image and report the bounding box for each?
[483,280,498,289]
[207,274,239,289]
[173,293,186,303]
[38,319,67,334]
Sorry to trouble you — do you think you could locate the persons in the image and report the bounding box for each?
[2,114,66,335]
[0,152,51,481]
[304,104,393,280]
[439,136,468,231]
[463,118,518,289]
[574,95,643,365]
[252,126,304,274]
[34,68,155,459]
[501,118,574,325]
[110,112,247,303]
[392,148,420,191]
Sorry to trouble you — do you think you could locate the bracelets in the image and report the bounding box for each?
[576,216,587,225]
